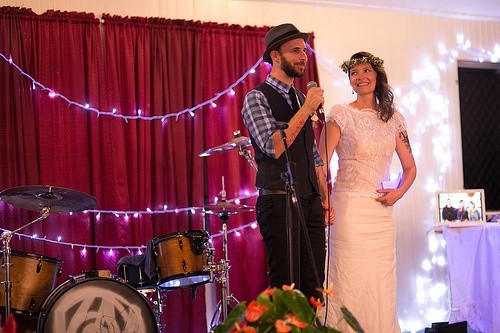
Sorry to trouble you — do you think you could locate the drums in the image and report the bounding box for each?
[116,253,159,293]
[0,249,63,318]
[144,229,217,291]
[36,270,162,333]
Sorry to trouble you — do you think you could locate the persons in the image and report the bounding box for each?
[442,198,480,221]
[315,53,417,333]
[241,23,336,313]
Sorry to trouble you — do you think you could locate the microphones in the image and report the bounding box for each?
[307,80,325,121]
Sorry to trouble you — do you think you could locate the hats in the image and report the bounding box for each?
[263,23,309,64]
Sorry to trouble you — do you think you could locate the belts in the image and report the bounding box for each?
[259,188,286,195]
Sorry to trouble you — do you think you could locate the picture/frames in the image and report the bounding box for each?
[435,189,486,228]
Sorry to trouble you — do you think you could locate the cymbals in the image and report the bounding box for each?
[0,185,98,212]
[197,136,253,158]
[188,200,256,215]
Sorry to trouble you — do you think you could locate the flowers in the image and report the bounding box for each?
[210,283,364,333]
[311,111,318,128]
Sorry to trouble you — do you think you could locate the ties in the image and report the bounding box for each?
[289,87,300,113]
